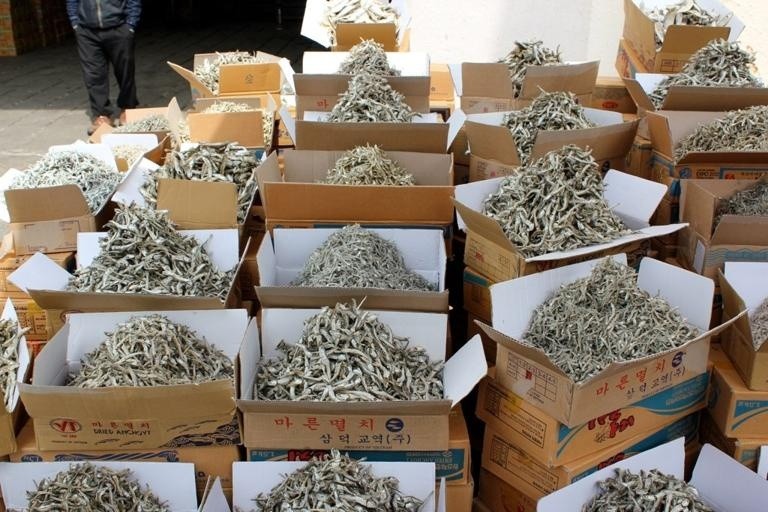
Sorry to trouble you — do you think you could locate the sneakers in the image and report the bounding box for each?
[119,109,126,126]
[88,116,116,135]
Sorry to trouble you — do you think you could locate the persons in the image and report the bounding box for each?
[62,0,147,137]
